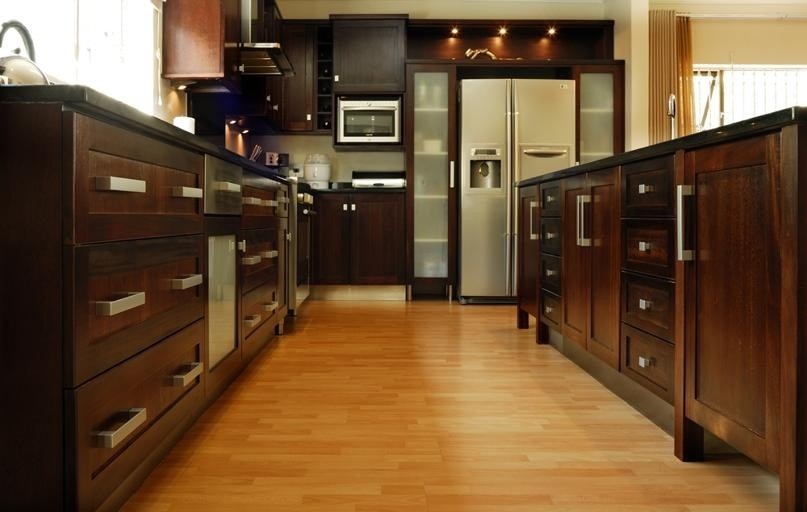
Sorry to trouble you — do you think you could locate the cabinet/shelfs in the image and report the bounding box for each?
[310,190,403,286]
[616,160,680,410]
[536,181,562,333]
[164,1,328,134]
[574,61,626,161]
[675,124,799,448]
[205,156,314,400]
[561,170,621,371]
[329,13,407,92]
[1,85,204,509]
[407,60,459,307]
[516,185,540,320]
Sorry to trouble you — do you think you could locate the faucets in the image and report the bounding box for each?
[667,93,678,140]
[0,20,36,62]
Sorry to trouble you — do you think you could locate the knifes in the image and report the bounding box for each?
[249,144,265,162]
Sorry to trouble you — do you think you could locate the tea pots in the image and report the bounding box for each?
[0,20,51,86]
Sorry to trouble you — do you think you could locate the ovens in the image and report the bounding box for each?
[292,182,318,308]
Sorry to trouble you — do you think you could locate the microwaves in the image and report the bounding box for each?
[337,95,402,142]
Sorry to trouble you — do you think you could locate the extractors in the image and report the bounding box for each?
[238,0,297,79]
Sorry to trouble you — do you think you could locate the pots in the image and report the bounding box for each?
[303,152,331,189]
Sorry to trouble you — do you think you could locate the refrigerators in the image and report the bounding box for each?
[459,78,576,297]
